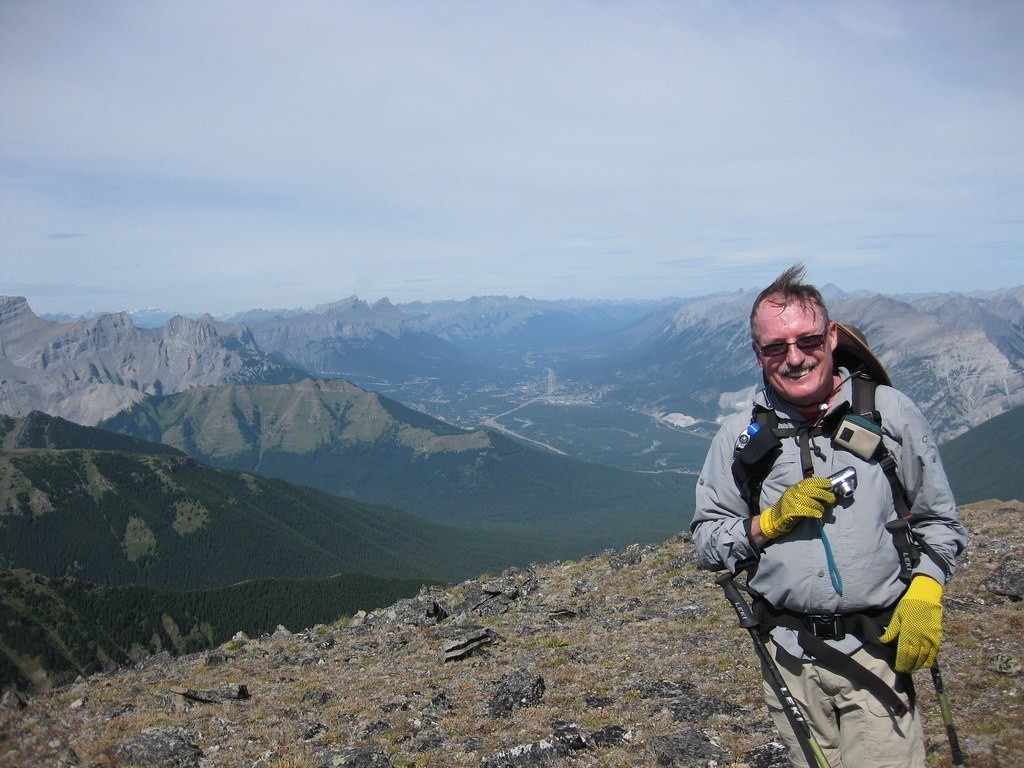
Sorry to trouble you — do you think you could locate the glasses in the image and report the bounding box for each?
[755,322,830,357]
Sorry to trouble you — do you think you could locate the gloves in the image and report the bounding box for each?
[760,477,837,537]
[878,576,943,673]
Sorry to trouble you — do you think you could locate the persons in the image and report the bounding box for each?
[690,265,969,767]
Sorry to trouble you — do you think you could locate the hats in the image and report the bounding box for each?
[834,322,893,386]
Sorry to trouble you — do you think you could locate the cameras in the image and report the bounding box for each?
[825,467,858,498]
